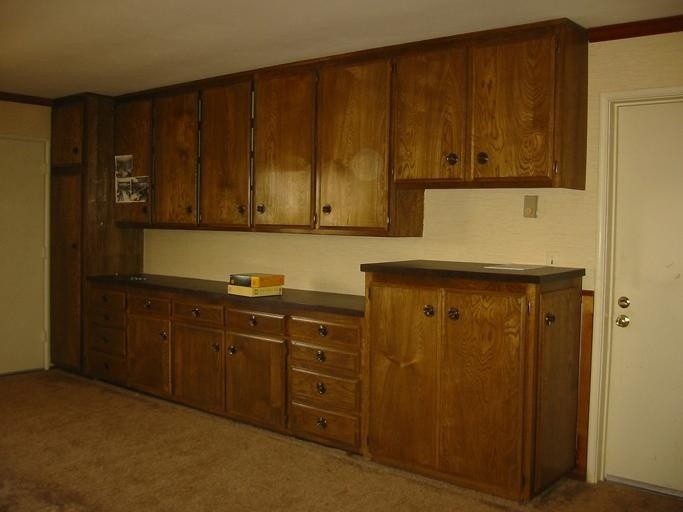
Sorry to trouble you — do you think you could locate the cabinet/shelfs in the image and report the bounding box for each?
[82,281,131,389]
[50,92,144,374]
[365,271,582,512]
[198,59,314,234]
[172,290,287,436]
[115,80,198,230]
[314,45,424,237]
[288,308,365,456]
[393,17,589,190]
[126,285,172,402]
